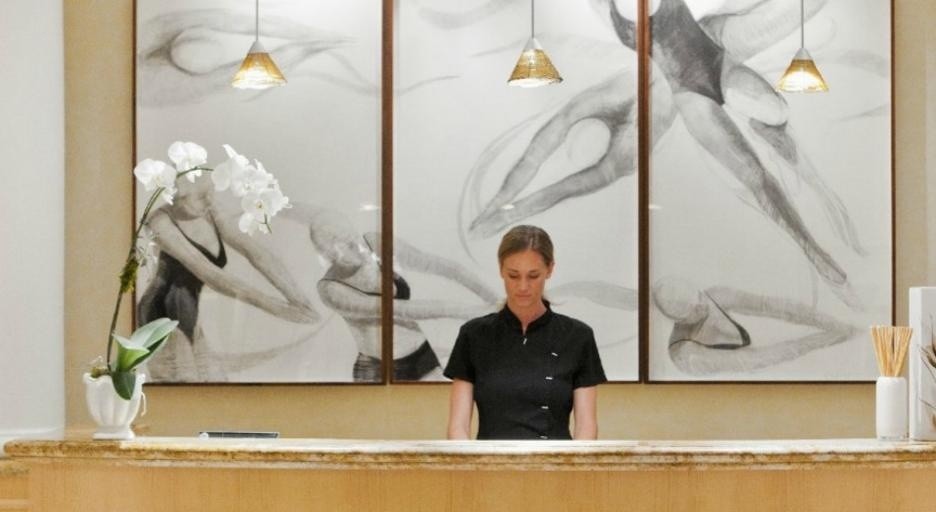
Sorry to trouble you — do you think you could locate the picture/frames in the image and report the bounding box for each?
[645,2,896,387]
[389,2,642,385]
[132,0,387,387]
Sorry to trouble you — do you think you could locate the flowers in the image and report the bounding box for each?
[86,141,290,403]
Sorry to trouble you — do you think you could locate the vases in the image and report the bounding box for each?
[80,366,148,443]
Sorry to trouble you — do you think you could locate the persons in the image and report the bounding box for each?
[443,227,607,441]
[468,2,823,249]
[610,0,884,314]
[310,207,501,381]
[652,277,868,373]
[137,164,322,383]
